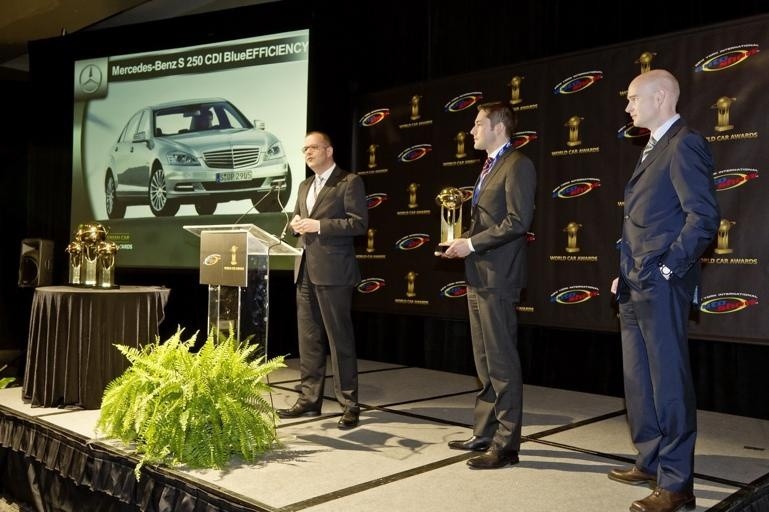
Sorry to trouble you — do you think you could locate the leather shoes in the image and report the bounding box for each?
[466,447,519,468]
[448,435,491,452]
[608,465,657,490]
[276,401,321,418]
[337,410,359,428]
[629,485,695,512]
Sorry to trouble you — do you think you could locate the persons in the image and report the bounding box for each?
[208,111,213,128]
[611,69,720,510]
[276,131,369,429]
[441,103,538,469]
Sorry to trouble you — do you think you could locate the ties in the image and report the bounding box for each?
[473,157,494,203]
[315,175,322,197]
[641,136,658,163]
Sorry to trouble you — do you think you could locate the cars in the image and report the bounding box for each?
[105,97,291,220]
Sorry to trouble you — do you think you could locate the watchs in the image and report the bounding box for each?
[662,266,671,275]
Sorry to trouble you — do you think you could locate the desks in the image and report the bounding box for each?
[22,286,171,407]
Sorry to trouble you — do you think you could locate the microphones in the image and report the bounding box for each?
[232,184,276,224]
[272,180,292,243]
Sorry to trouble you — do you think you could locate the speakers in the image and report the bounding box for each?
[18,238,54,288]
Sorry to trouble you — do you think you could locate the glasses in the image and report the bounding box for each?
[301,144,319,153]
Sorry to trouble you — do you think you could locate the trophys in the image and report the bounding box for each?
[64,223,120,289]
[435,187,464,253]
